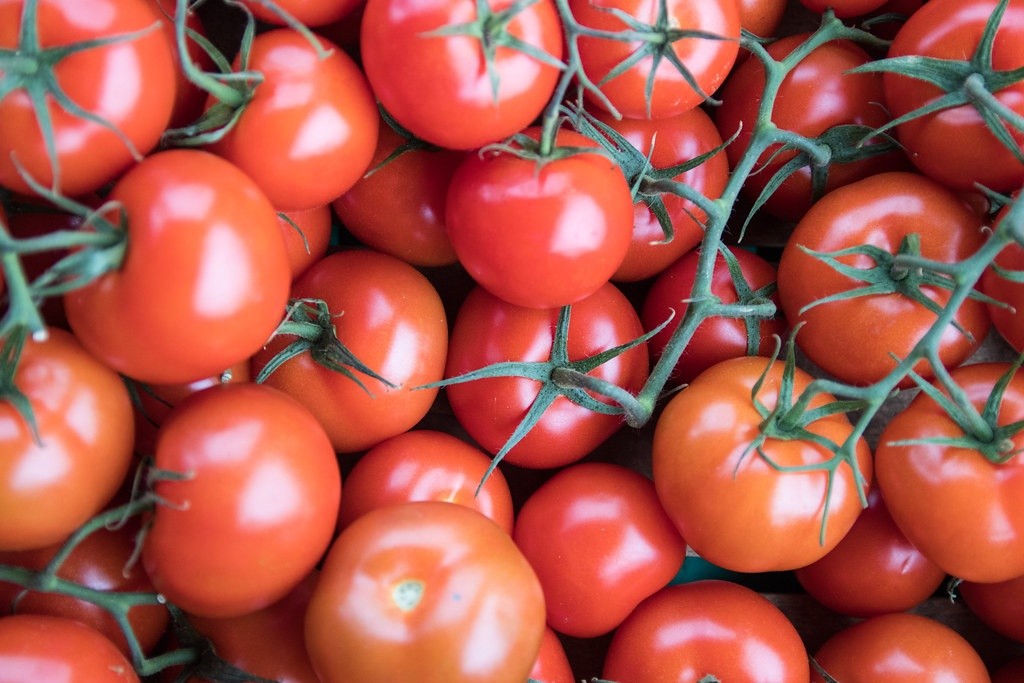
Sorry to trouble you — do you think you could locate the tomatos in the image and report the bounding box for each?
[0,0,1024,683]
